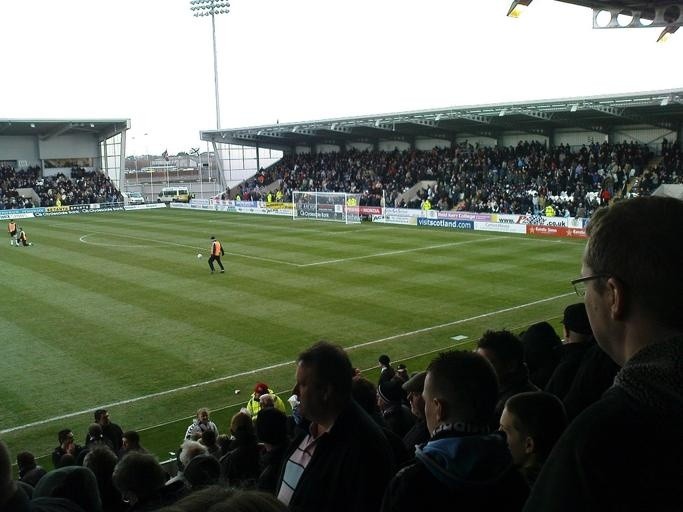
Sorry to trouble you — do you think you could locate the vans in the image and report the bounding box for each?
[156,187,191,203]
[124,191,145,204]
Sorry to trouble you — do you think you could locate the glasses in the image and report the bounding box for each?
[571,275,601,296]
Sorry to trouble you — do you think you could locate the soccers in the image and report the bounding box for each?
[198,253,202,258]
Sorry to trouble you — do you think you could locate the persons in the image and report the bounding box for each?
[8,219,17,245]
[516,196,681,511]
[0,160,124,212]
[16,229,33,246]
[208,236,225,274]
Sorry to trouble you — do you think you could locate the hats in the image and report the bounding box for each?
[255,384,267,393]
[184,455,221,484]
[560,304,590,334]
[378,371,426,405]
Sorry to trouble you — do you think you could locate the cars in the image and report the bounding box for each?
[124,167,155,175]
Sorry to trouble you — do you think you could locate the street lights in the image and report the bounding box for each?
[188,0,232,153]
